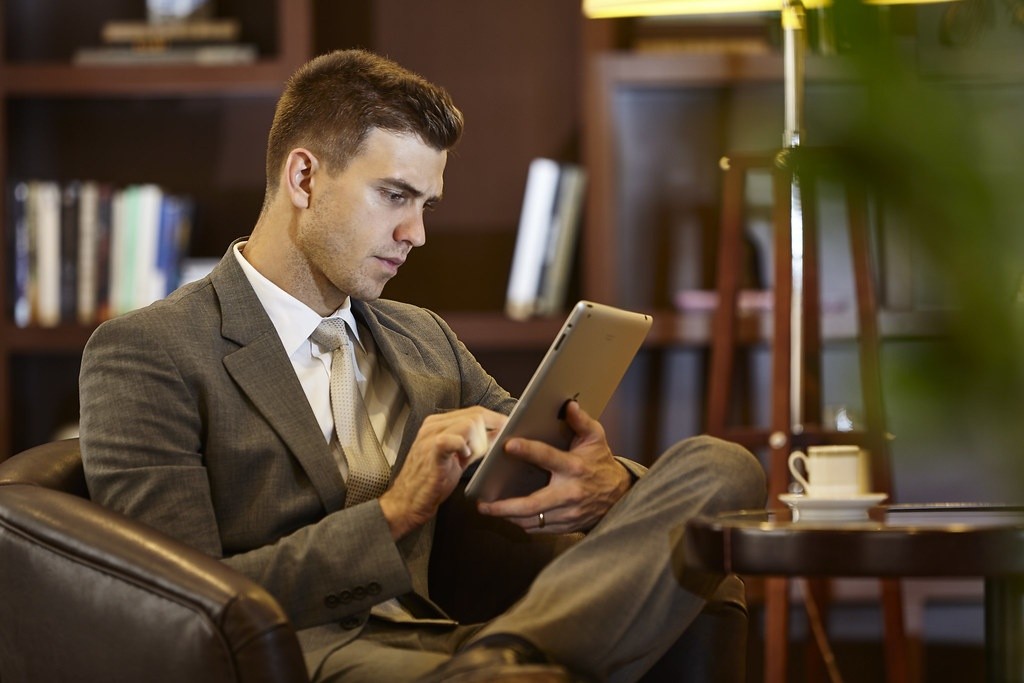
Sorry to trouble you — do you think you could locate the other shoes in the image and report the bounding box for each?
[414,639,569,683]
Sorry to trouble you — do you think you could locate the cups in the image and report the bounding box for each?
[789,446,860,493]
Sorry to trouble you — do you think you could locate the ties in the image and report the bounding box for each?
[311,318,459,628]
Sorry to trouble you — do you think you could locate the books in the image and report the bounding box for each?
[14,180,221,329]
[885,503,1023,526]
[76,0,255,70]
[505,155,583,321]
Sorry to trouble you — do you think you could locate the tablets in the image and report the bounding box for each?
[465,300,654,502]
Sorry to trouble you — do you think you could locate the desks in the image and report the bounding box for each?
[687,501,1023,683]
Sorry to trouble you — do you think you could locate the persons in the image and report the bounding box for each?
[79,48,770,683]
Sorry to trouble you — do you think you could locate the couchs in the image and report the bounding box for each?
[0,438,309,682]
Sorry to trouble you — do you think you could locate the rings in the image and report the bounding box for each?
[539,513,544,527]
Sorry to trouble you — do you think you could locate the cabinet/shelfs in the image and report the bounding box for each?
[0,0,948,509]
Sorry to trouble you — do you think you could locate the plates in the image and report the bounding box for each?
[778,494,888,520]
